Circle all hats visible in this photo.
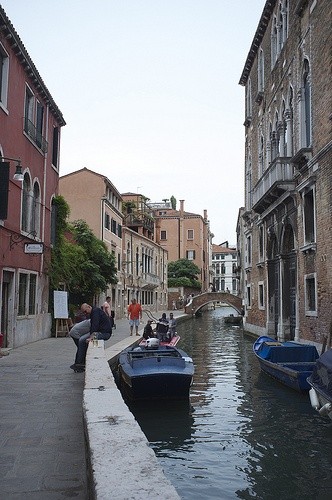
[130,298,136,301]
[148,320,153,322]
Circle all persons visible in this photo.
[70,319,90,372]
[126,299,143,336]
[143,312,176,350]
[70,303,112,372]
[170,285,233,310]
[103,296,114,337]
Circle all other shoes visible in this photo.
[70,364,86,373]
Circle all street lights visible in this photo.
[0,153,25,182]
[121,260,143,317]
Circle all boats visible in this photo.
[224,313,242,324]
[252,333,321,392]
[305,346,332,421]
[117,337,195,397]
[138,330,181,347]
